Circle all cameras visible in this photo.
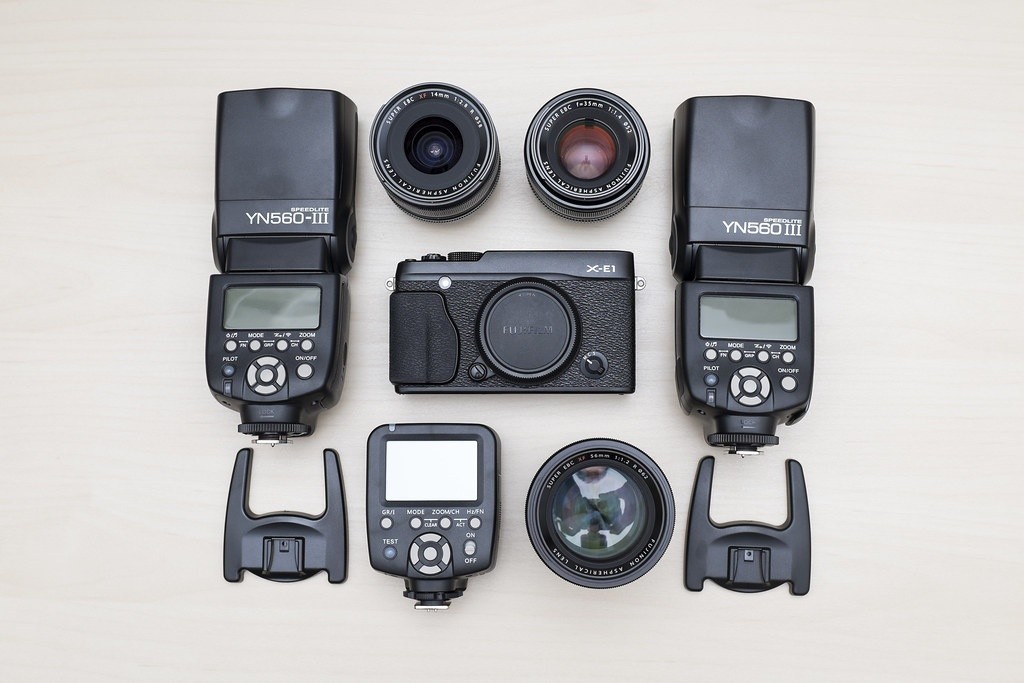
[387,251,639,396]
